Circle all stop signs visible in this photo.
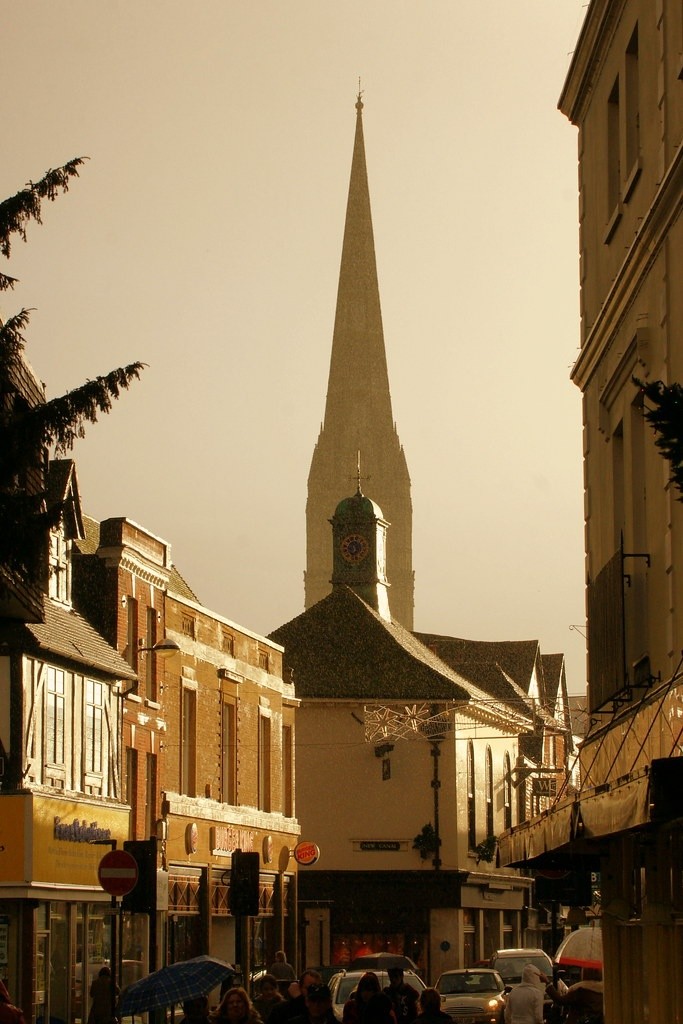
[97,850,138,896]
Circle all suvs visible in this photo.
[434,969,513,1024]
[328,967,448,1024]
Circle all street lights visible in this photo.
[90,839,118,1024]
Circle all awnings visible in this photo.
[495,755,683,869]
[553,926,603,971]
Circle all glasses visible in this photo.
[359,986,376,993]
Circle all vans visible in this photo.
[489,948,554,1018]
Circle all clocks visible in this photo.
[341,535,370,563]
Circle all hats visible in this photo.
[359,973,378,987]
[307,983,330,999]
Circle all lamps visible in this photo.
[139,638,180,657]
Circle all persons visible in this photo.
[503,963,604,1024]
[478,975,492,989]
[87,967,122,1024]
[454,978,468,989]
[179,949,342,1024]
[342,972,456,1024]
[0,977,27,1024]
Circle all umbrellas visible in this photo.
[116,955,235,1018]
[347,952,419,990]
